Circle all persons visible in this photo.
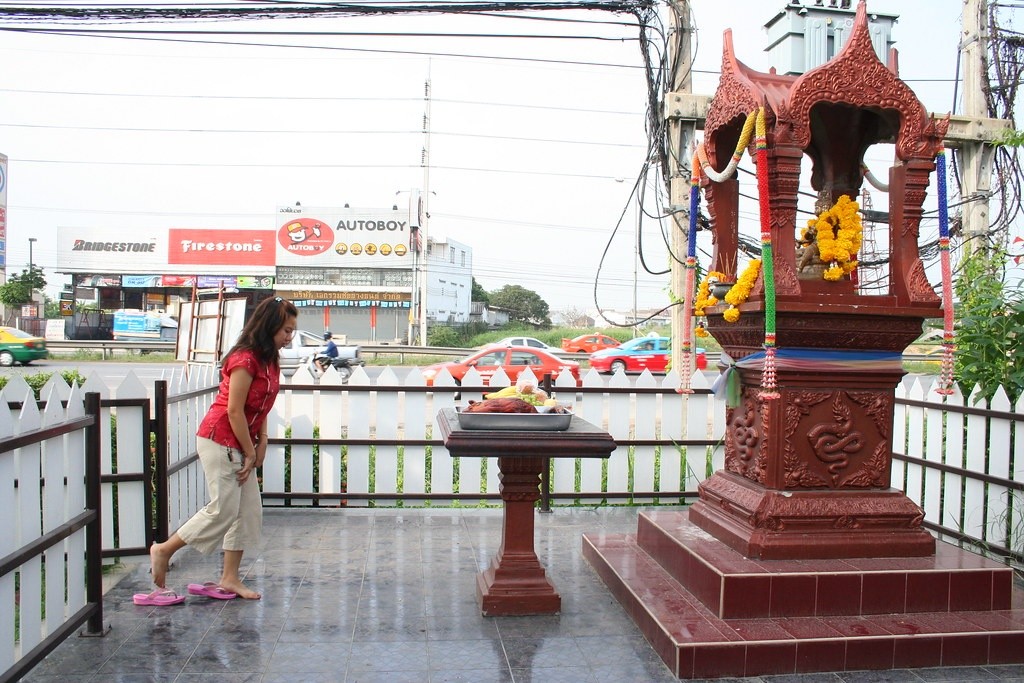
[494,352,505,365]
[637,343,652,351]
[150,297,298,599]
[313,331,338,376]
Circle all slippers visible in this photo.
[133,587,186,606]
[187,581,237,599]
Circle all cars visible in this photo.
[561,332,623,363]
[590,332,708,376]
[277,329,359,370]
[478,334,567,365]
[420,342,583,403]
[0,325,50,367]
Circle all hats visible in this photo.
[324,332,330,339]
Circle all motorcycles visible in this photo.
[295,347,357,384]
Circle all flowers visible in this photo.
[801,194,864,281]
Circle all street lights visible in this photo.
[29,238,38,300]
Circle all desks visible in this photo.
[436,408,617,616]
